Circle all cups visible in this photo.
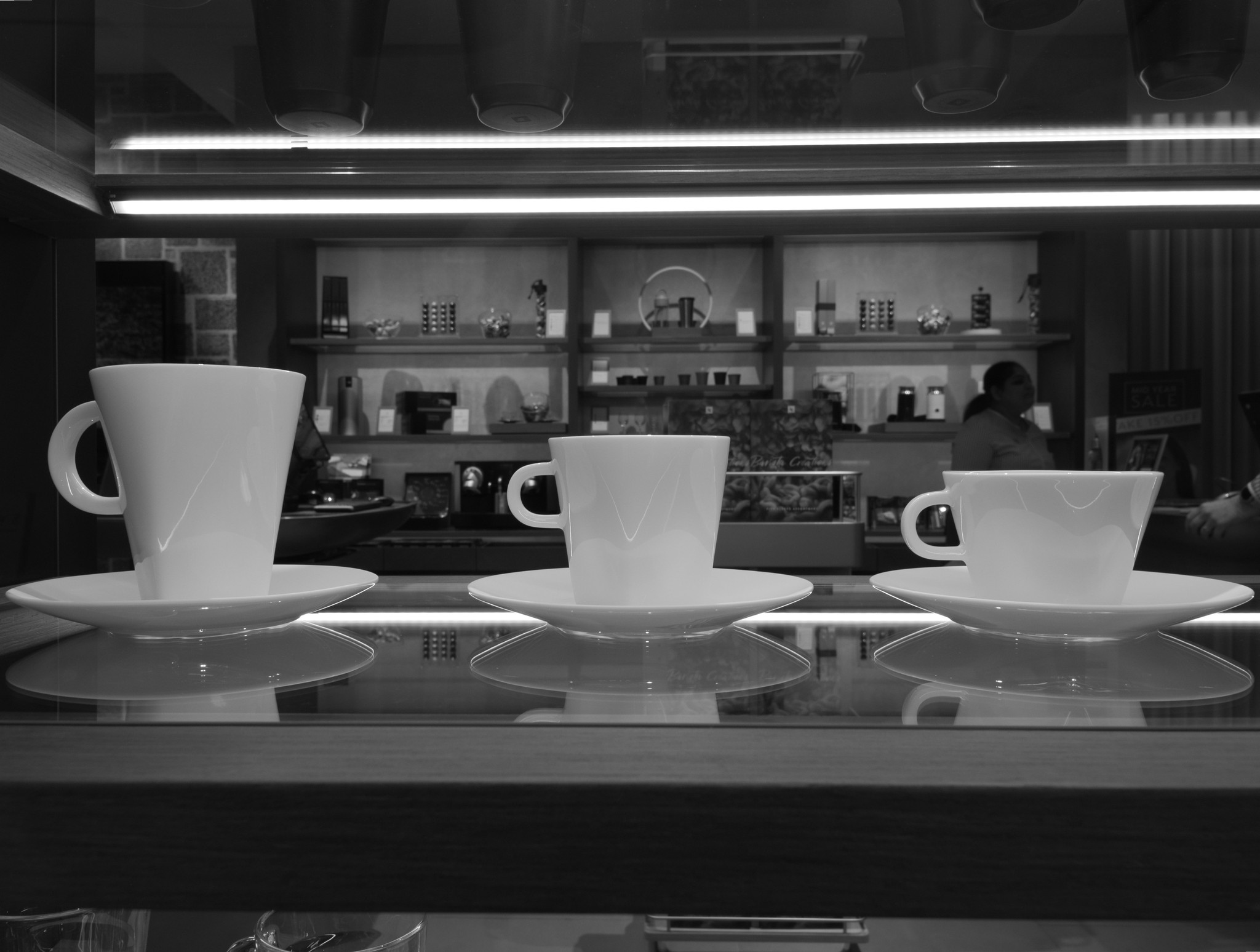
[46,364,306,603]
[93,688,283,724]
[616,372,740,386]
[900,469,1163,604]
[506,438,730,608]
[511,691,721,724]
[901,682,1147,727]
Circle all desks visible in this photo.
[0,582,1260,951]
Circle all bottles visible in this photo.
[927,386,944,420]
[897,385,914,420]
[337,376,360,436]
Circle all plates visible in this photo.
[872,624,1254,702]
[469,627,816,699]
[6,620,375,704]
[869,566,1255,636]
[5,563,380,639]
[466,569,814,639]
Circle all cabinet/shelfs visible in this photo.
[280,240,1077,528]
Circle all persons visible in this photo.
[951,361,1054,473]
[1185,474,1260,540]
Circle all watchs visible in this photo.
[1240,484,1257,505]
